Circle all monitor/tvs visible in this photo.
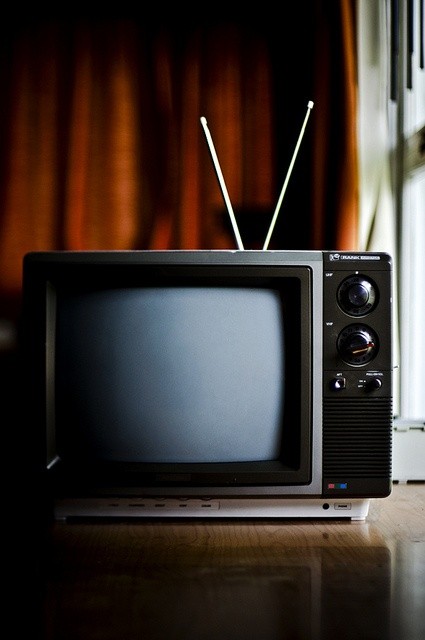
[23,101,395,523]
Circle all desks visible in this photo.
[0,518,392,639]
